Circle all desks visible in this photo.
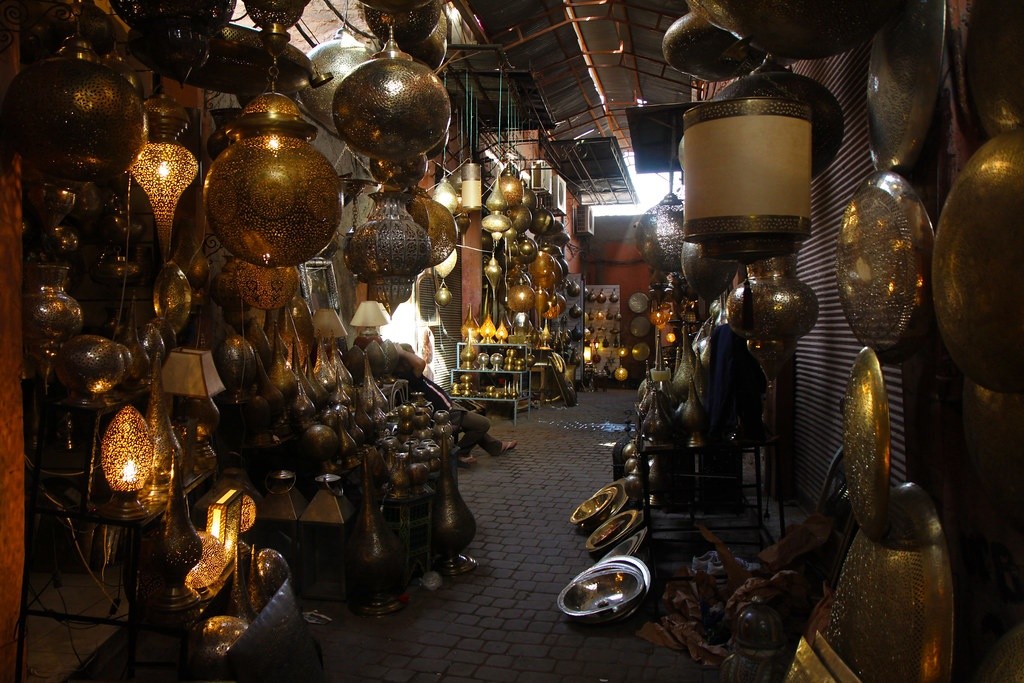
[643,431,781,564]
[16,462,214,682]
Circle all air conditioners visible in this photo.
[545,173,566,217]
[574,204,595,236]
[531,160,554,196]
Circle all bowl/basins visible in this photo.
[557,478,651,622]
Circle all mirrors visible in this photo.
[298,257,348,358]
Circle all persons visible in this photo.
[394,344,517,469]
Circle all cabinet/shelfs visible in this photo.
[451,342,534,427]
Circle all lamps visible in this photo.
[633,1,1024,683]
[484,73,631,382]
[0,0,481,682]
